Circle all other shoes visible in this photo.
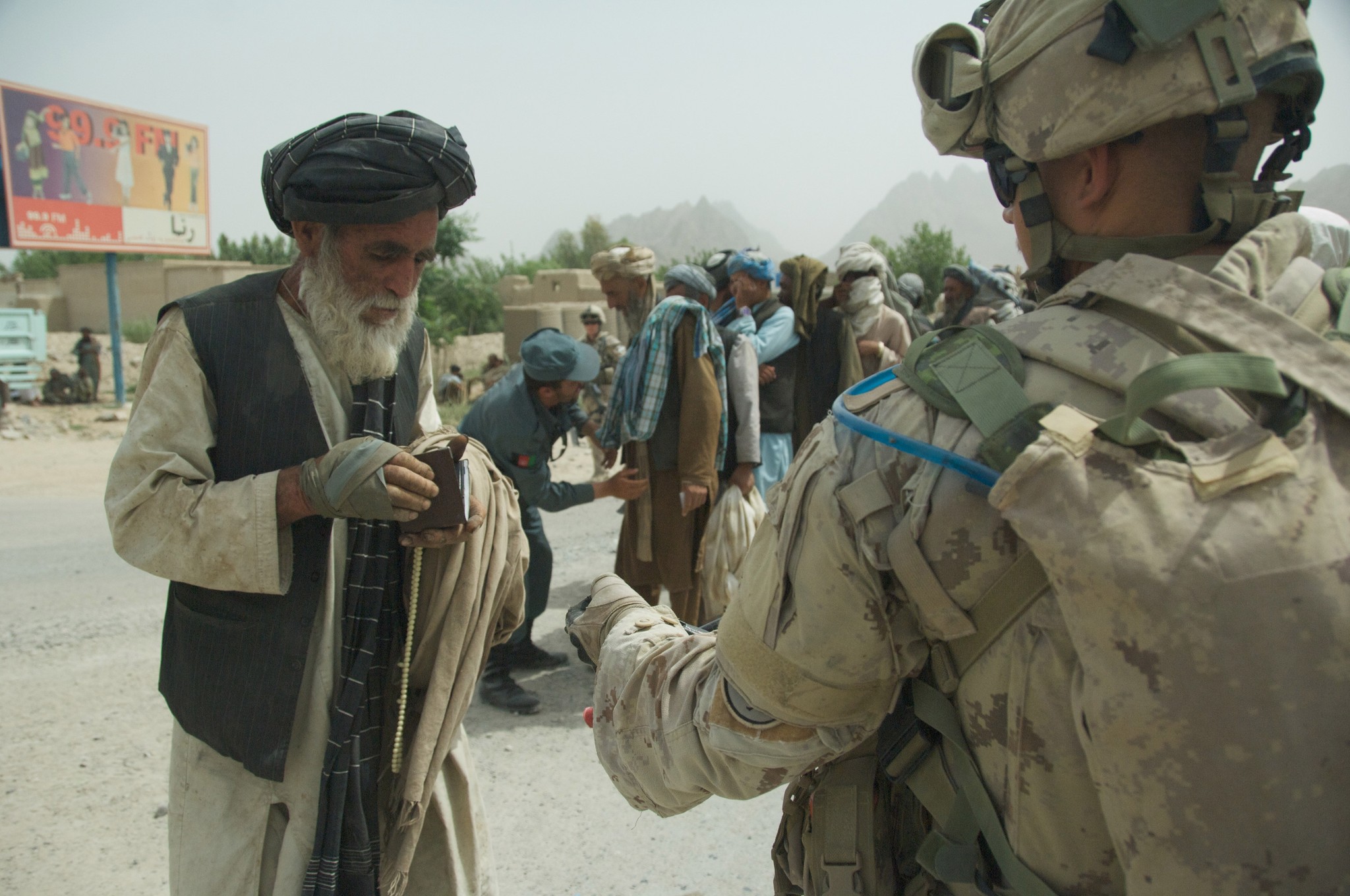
[507,640,570,672]
[478,678,543,716]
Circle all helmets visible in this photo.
[913,0,1325,164]
[579,305,606,326]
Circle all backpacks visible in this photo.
[894,312,1350,896]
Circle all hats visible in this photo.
[944,261,980,288]
[260,110,477,239]
[665,263,718,299]
[520,326,602,383]
[590,245,655,279]
[727,249,774,281]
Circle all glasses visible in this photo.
[582,313,598,319]
[982,137,1034,208]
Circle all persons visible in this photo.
[583,240,1044,626]
[70,327,102,403]
[562,0,1349,896]
[103,137,503,896]
[42,368,75,406]
[457,327,650,714]
[434,353,508,408]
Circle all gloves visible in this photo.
[563,572,650,673]
